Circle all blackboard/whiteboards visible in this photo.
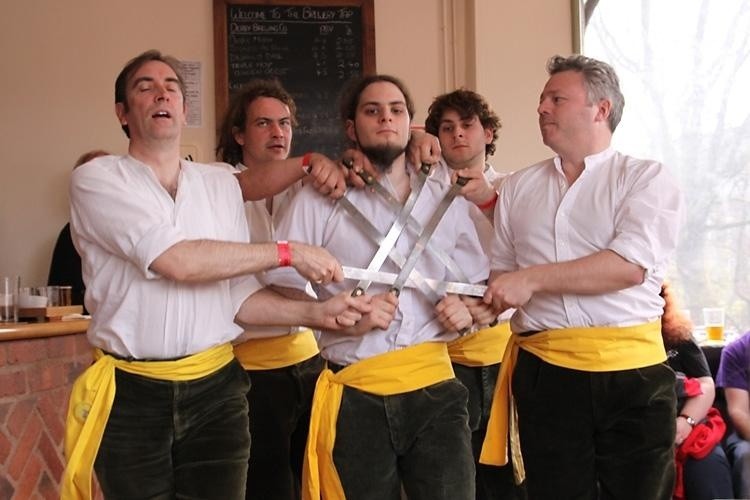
[214,1,377,166]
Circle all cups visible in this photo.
[47,286,59,306]
[1,275,20,323]
[60,286,71,305]
[705,308,725,340]
[31,288,39,296]
[24,287,31,296]
[39,287,47,296]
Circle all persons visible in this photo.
[68,50,372,500]
[47,149,112,316]
[263,76,491,500]
[215,81,379,500]
[715,329,750,499]
[462,54,683,500]
[410,89,514,494]
[660,284,732,499]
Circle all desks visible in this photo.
[0,316,105,500]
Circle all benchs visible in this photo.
[701,345,726,410]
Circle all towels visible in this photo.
[62,313,92,320]
